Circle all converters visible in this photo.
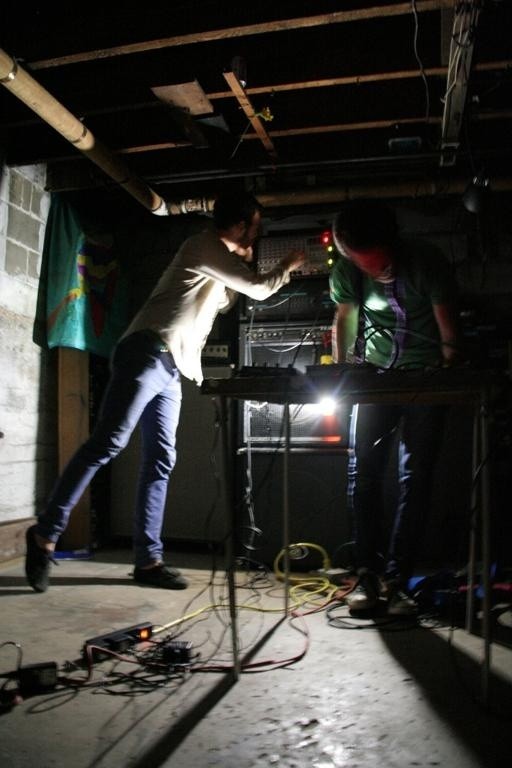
[16,660,57,694]
[162,640,194,663]
[315,567,351,586]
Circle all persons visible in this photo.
[319,195,464,616]
[20,187,308,598]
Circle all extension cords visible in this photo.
[83,621,153,659]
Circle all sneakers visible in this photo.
[26,524,58,592]
[348,585,418,614]
[134,563,186,590]
